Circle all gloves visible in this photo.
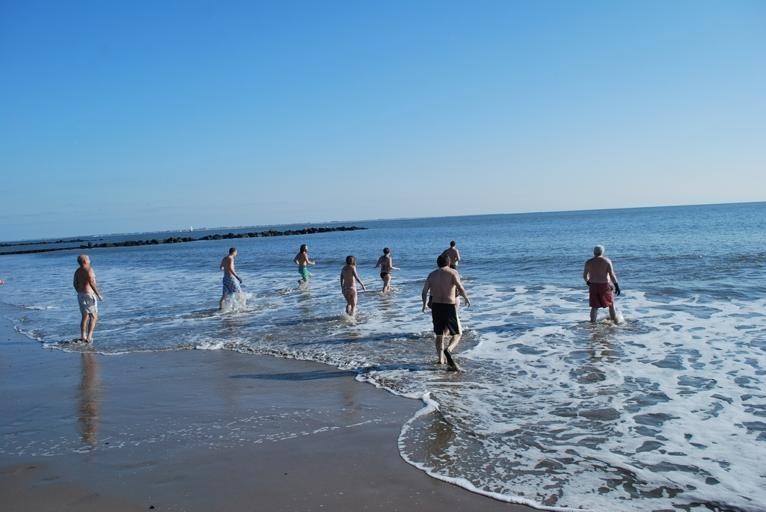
[614,283,620,296]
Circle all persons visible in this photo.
[76,346,106,448]
[583,244,621,323]
[440,241,460,270]
[449,263,464,311]
[73,254,104,344]
[293,243,316,286]
[374,247,401,293]
[220,247,242,310]
[421,253,470,372]
[339,255,366,319]
